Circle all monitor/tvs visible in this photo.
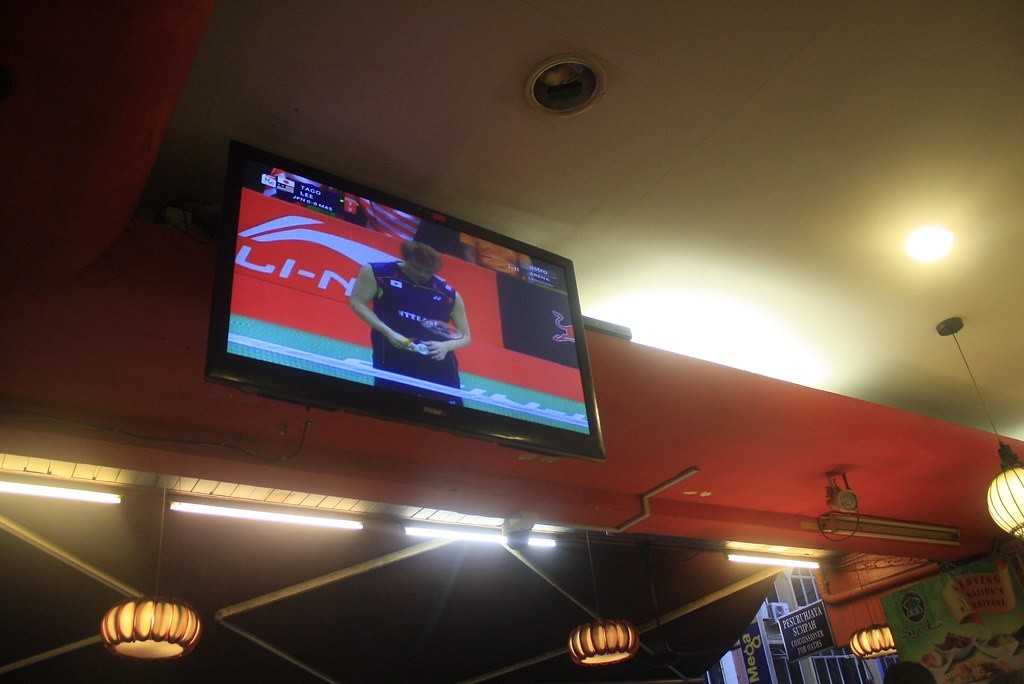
[203,140,607,463]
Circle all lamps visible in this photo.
[100,473,202,662]
[846,551,898,662]
[936,315,1024,540]
[567,528,638,670]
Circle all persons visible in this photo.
[348,238,470,409]
[457,230,536,290]
